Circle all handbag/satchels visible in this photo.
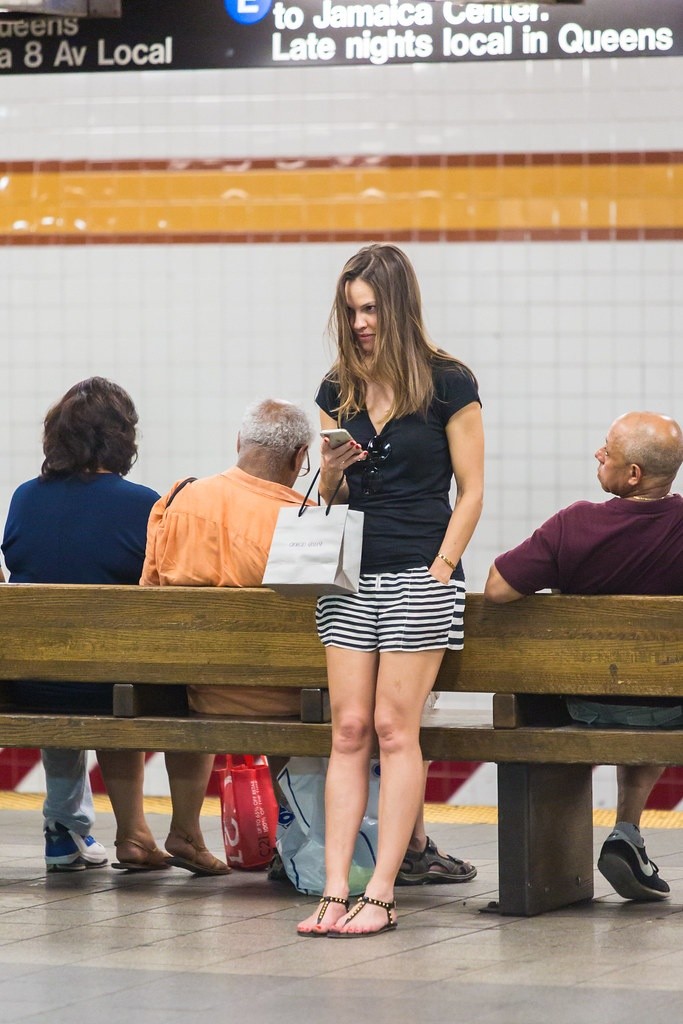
[215,752,279,872]
[260,466,365,595]
[275,756,380,898]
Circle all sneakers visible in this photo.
[598,830,670,900]
[44,822,108,870]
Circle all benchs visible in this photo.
[0,582,683,922]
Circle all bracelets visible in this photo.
[436,553,457,571]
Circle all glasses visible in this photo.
[361,435,392,494]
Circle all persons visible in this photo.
[484,411,682,900]
[138,398,477,885]
[0,376,232,876]
[296,244,484,939]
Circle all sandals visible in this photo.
[396,833,477,883]
[113,836,176,870]
[164,825,233,875]
[297,898,398,939]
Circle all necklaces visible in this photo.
[634,492,671,501]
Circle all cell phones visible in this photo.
[320,429,363,456]
